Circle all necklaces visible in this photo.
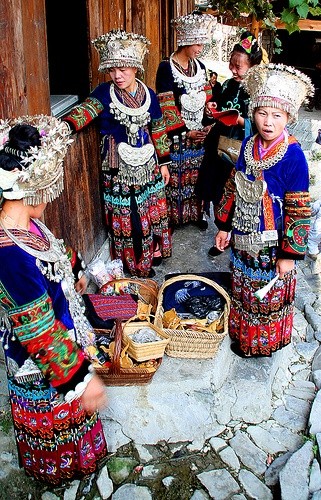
[232,130,288,234]
[170,51,205,131]
[0,207,89,344]
[109,78,155,185]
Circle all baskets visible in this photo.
[121,314,170,362]
[154,275,231,359]
[98,278,159,325]
[92,317,163,387]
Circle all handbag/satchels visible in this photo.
[217,117,254,165]
[81,292,141,329]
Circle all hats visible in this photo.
[239,63,315,127]
[0,113,75,206]
[170,13,217,46]
[91,30,151,74]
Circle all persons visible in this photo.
[193,31,263,258]
[154,11,218,236]
[209,72,221,99]
[60,29,173,278]
[0,114,107,486]
[214,63,312,358]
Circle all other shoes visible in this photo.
[146,268,156,278]
[208,244,230,258]
[198,219,208,232]
